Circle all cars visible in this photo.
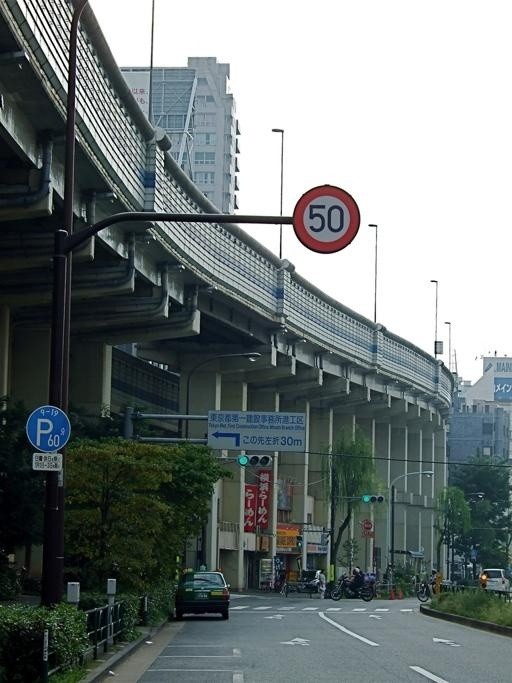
[174,570,231,618]
[297,570,326,593]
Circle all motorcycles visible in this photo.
[330,573,375,601]
[415,581,434,602]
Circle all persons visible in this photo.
[430,569,438,594]
[347,566,363,597]
[481,570,489,593]
[318,569,326,600]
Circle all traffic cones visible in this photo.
[383,590,403,600]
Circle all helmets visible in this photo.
[353,567,360,575]
[431,568,437,574]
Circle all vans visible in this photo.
[479,568,510,593]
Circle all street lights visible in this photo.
[430,280,450,370]
[272,129,284,259]
[387,470,434,568]
[368,224,377,322]
[452,492,485,582]
[185,352,263,438]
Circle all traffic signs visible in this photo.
[292,185,361,254]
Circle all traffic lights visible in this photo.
[236,455,273,468]
[361,494,385,504]
